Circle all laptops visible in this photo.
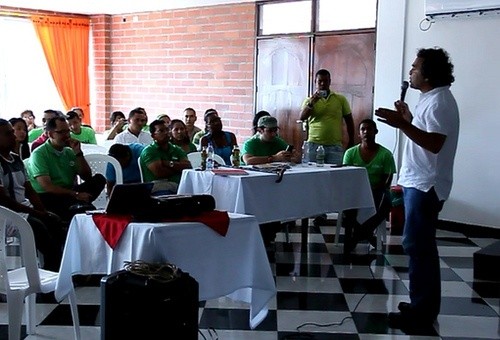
[86,182,154,215]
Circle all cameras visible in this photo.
[317,90,327,97]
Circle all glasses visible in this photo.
[54,129,70,135]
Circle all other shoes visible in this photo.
[369,235,384,249]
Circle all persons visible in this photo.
[0,106,301,274]
[375,46,460,331]
[300,69,354,165]
[342,119,396,259]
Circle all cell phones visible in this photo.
[286,144,294,152]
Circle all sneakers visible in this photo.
[398,302,438,320]
[389,312,439,336]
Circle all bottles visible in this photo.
[232,145,240,168]
[201,146,206,171]
[302,141,310,167]
[315,146,325,168]
[206,142,214,170]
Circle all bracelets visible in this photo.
[75,192,79,199]
[307,105,312,110]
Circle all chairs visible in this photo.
[0,204,83,340]
[137,156,167,200]
[334,207,387,251]
[186,152,226,169]
[76,153,125,210]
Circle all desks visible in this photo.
[52,208,277,340]
[174,160,379,270]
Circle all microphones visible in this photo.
[401,80,408,101]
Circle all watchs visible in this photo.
[169,161,174,168]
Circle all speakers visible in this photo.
[99,270,199,340]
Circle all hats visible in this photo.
[257,116,280,130]
